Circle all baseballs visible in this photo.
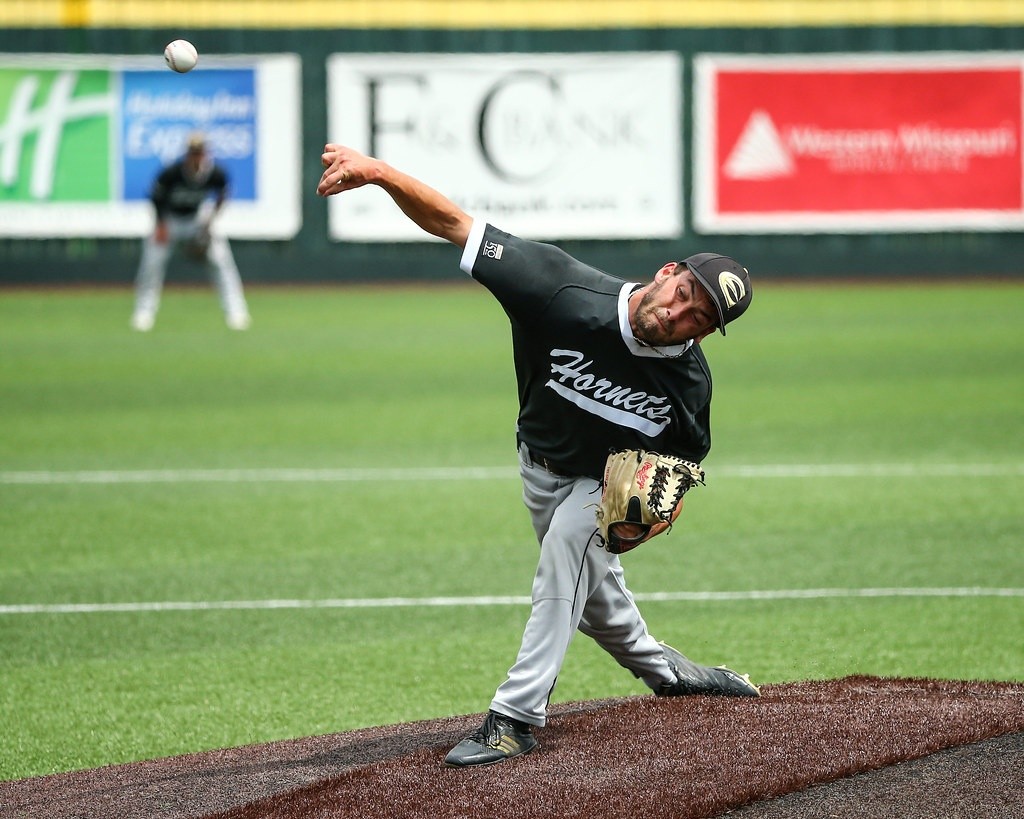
[164,39,199,74]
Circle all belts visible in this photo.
[517,432,604,476]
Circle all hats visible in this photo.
[680,253,752,336]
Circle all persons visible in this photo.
[134,138,249,329]
[317,144,763,766]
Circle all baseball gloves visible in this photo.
[582,447,708,555]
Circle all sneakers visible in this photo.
[654,640,761,697]
[444,710,538,767]
